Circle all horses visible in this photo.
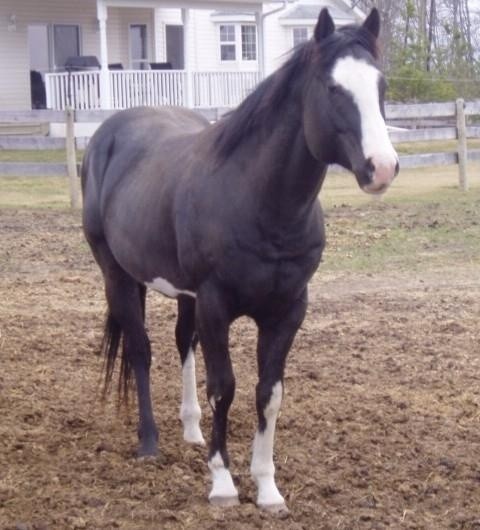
[81,6,400,520]
